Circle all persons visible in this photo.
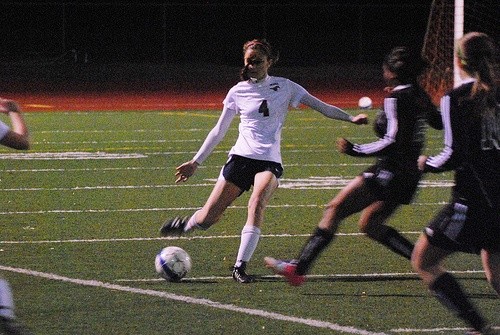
[263,49,444,286]
[0,100,29,335]
[160,39,368,283]
[411,32,500,335]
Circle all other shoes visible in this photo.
[0,316,29,335]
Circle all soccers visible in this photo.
[358,96,373,109]
[154,246,192,282]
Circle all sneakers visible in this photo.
[232,260,254,283]
[160,216,186,233]
[264,255,304,286]
